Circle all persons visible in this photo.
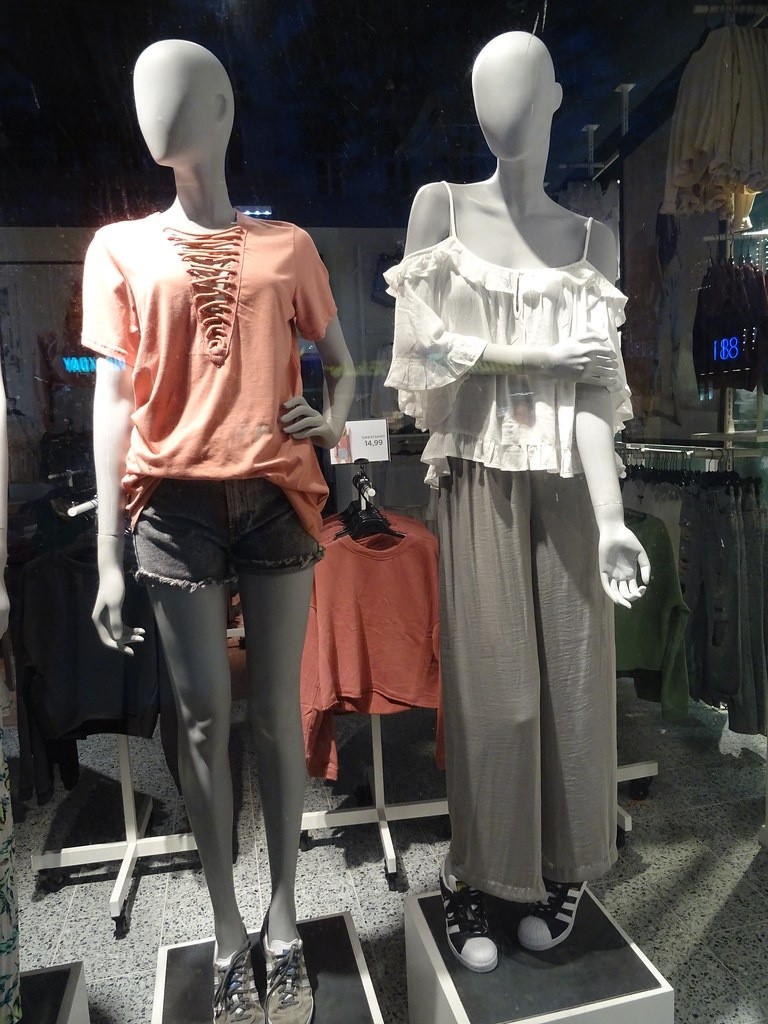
[383,30,652,974]
[79,39,357,1024]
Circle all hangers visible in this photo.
[333,476,405,542]
[703,232,764,279]
[692,0,768,40]
[618,448,762,491]
[41,470,137,560]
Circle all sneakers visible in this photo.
[518,871,588,951]
[259,905,313,1024]
[210,920,265,1024]
[439,857,497,973]
